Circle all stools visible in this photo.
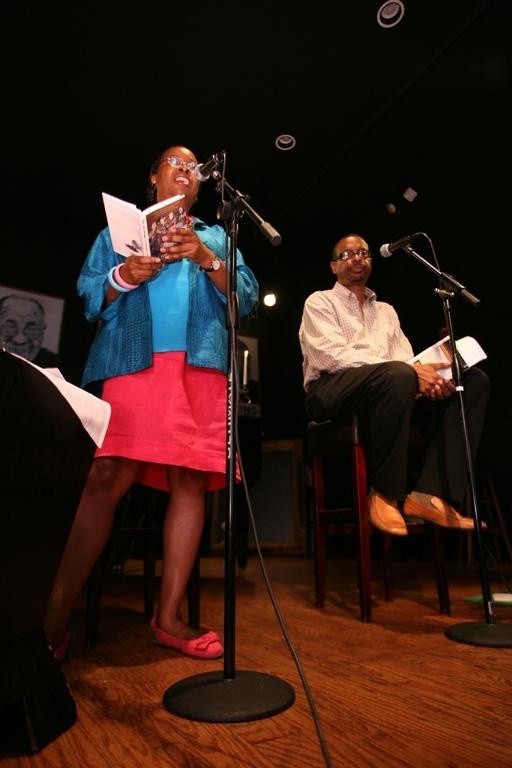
[303,410,454,624]
[79,509,204,644]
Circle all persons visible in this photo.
[44,145,259,659]
[0,294,47,361]
[298,232,489,538]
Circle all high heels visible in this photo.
[49,606,85,661]
[150,616,224,659]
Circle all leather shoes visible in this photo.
[368,491,408,537]
[404,493,486,531]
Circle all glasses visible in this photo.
[158,157,200,172]
[334,249,372,261]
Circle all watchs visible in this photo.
[199,254,221,273]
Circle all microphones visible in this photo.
[194,153,221,183]
[379,236,412,258]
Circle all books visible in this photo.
[414,336,465,398]
[98,190,188,266]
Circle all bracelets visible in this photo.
[109,266,131,293]
[114,263,139,290]
